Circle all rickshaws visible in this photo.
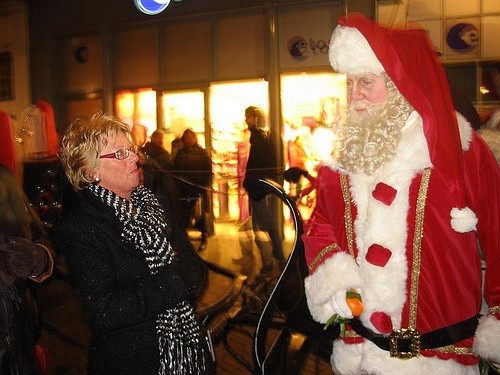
[0,100,317,374]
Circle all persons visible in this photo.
[288,133,312,220]
[0,163,56,375]
[301,12,500,375]
[141,128,212,249]
[232,107,286,271]
[57,117,219,375]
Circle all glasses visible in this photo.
[99,144,138,160]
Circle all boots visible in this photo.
[198,213,209,251]
